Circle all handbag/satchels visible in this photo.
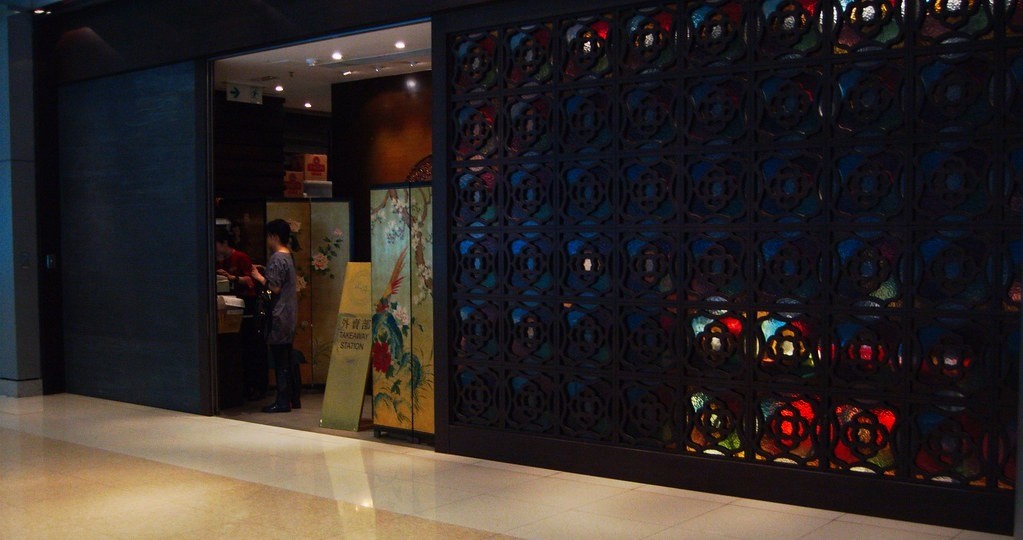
[238,281,281,336]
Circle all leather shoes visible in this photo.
[290,399,301,408]
[262,403,291,412]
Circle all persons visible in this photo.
[251,219,302,413]
[214,226,256,289]
